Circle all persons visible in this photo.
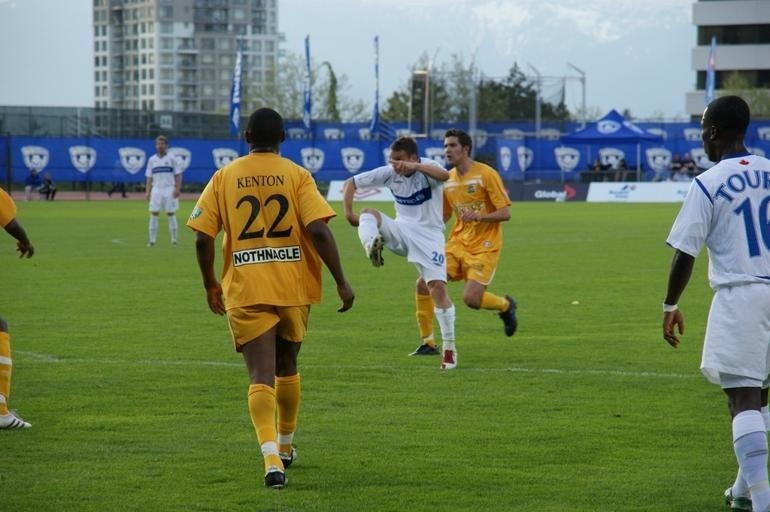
[409,126,519,359]
[0,183,37,430]
[341,135,461,373]
[190,104,355,493]
[38,172,58,201]
[142,133,184,248]
[107,181,132,199]
[589,152,700,183]
[24,167,41,201]
[658,91,770,512]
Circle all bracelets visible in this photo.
[662,301,679,314]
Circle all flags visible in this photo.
[225,34,244,137]
[703,36,718,104]
[301,33,314,130]
[368,36,381,137]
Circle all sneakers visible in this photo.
[724,487,753,511]
[368,235,385,266]
[0,412,31,429]
[409,344,439,356]
[441,350,457,369]
[499,295,517,336]
[264,447,297,489]
